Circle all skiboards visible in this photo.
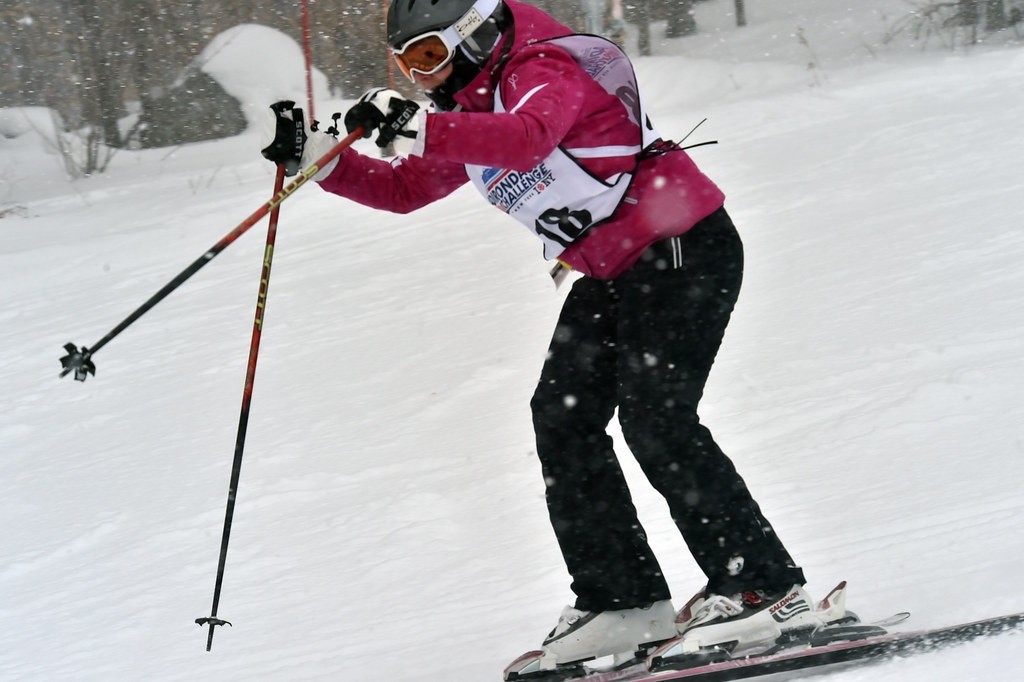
[501,577,1024,682]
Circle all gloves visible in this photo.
[344,86,420,160]
[260,98,340,181]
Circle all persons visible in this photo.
[259,1,815,666]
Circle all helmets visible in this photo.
[387,1,508,65]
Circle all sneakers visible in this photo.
[680,582,816,645]
[541,598,677,663]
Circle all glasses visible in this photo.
[392,32,453,83]
